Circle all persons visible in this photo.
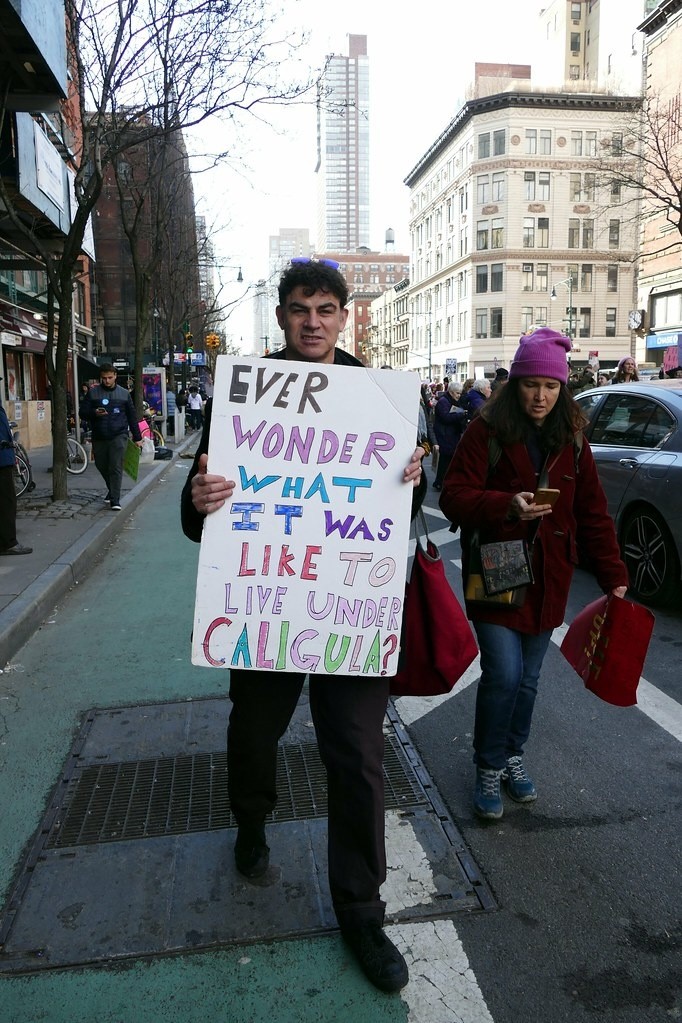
[437,329,629,819]
[166,384,209,437]
[416,368,511,492]
[80,382,92,431]
[78,362,143,511]
[0,404,34,556]
[180,262,428,991]
[565,358,610,410]
[608,357,641,386]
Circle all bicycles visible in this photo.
[140,412,165,454]
[65,435,89,475]
[8,421,37,498]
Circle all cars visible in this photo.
[564,379,682,607]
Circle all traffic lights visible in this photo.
[206,335,211,347]
[210,334,216,350]
[215,336,220,346]
[361,344,367,353]
[185,334,194,354]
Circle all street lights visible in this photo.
[549,268,582,371]
[151,288,162,367]
[179,263,245,391]
[396,307,433,383]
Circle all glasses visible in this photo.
[290,256,340,271]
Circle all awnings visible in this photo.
[0,306,81,359]
[77,353,101,379]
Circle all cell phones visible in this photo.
[532,489,560,507]
[99,408,106,413]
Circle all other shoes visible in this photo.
[432,482,442,490]
[105,491,121,510]
[1,542,33,555]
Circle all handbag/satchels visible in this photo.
[463,539,533,606]
[396,538,480,697]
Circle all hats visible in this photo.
[618,356,637,370]
[508,328,572,383]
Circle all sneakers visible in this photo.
[474,760,503,820]
[234,817,269,878]
[504,755,538,802]
[347,929,409,991]
